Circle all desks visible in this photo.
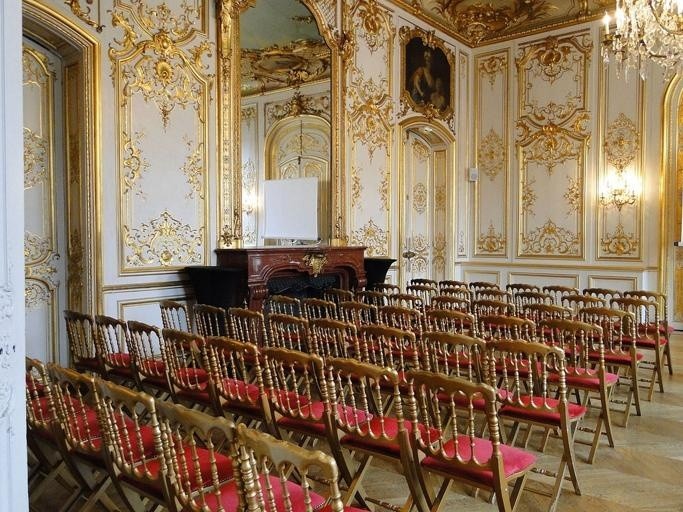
[178,246,398,347]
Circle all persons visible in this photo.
[408,50,435,105]
[428,78,446,112]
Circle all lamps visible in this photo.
[600,0,683,81]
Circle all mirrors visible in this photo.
[216,0,349,250]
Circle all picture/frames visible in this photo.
[398,24,456,121]
